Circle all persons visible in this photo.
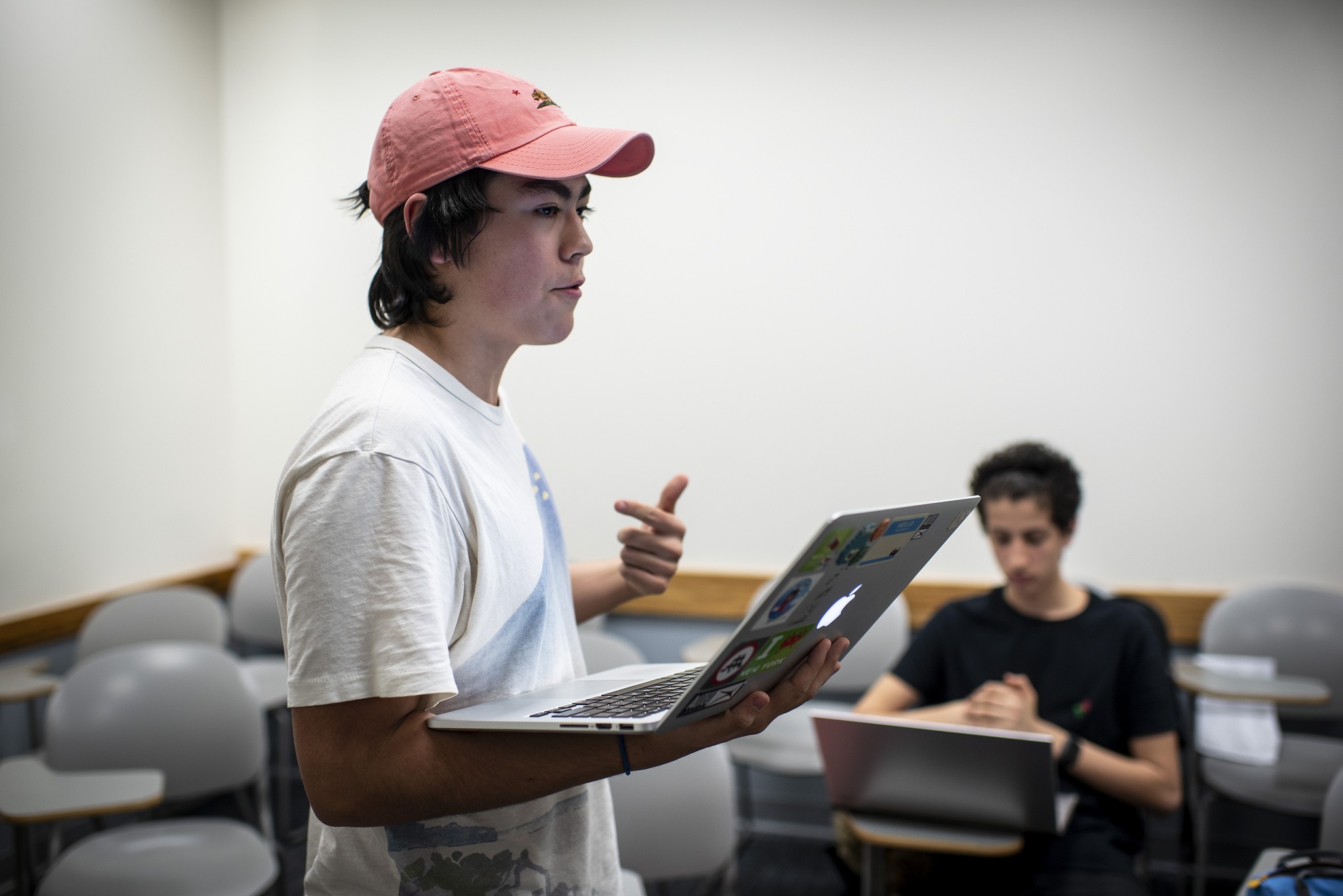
[271,65,851,895]
[853,443,1184,896]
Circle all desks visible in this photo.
[0,653,58,750]
[830,802,1024,896]
[0,754,168,896]
[1173,658,1333,706]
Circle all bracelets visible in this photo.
[1054,732,1080,772]
[617,734,631,775]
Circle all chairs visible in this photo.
[735,578,912,847]
[226,554,294,710]
[1182,583,1343,896]
[75,584,231,668]
[578,608,742,896]
[31,643,283,896]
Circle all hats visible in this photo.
[367,67,655,228]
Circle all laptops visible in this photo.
[811,710,1079,834]
[428,494,980,731]
